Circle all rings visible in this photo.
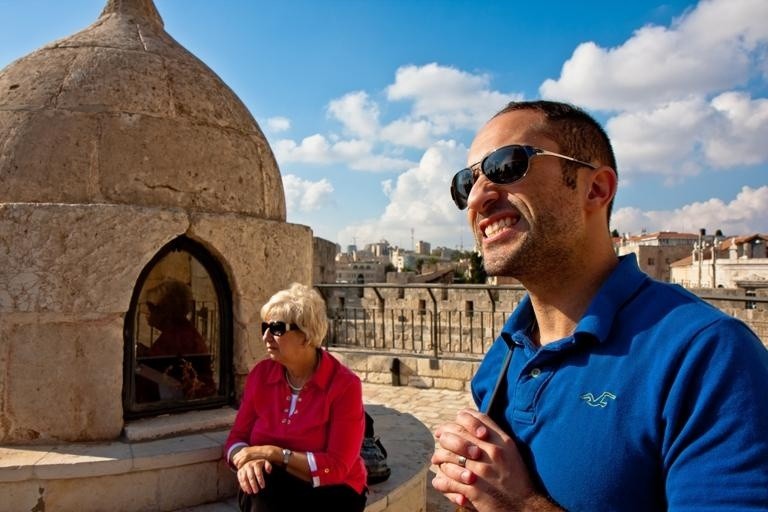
[457,455,468,470]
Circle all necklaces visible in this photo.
[285,352,321,391]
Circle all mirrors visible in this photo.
[122,234,234,419]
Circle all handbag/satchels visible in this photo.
[360,435,391,486]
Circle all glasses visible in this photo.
[261,319,299,337]
[145,300,166,313]
[450,142,599,214]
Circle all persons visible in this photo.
[430,100,768,512]
[136,278,218,406]
[222,280,371,512]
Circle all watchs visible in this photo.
[279,448,291,468]
